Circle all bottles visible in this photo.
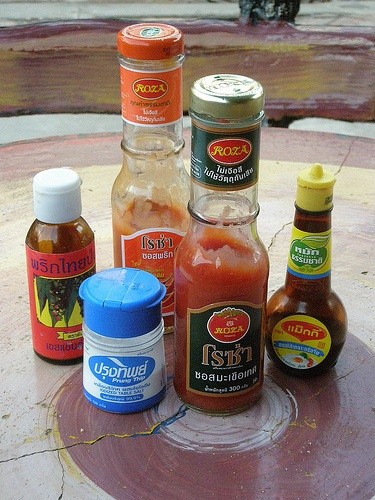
[265,162,348,378]
[110,23,191,336]
[24,168,96,364]
[173,72,270,415]
